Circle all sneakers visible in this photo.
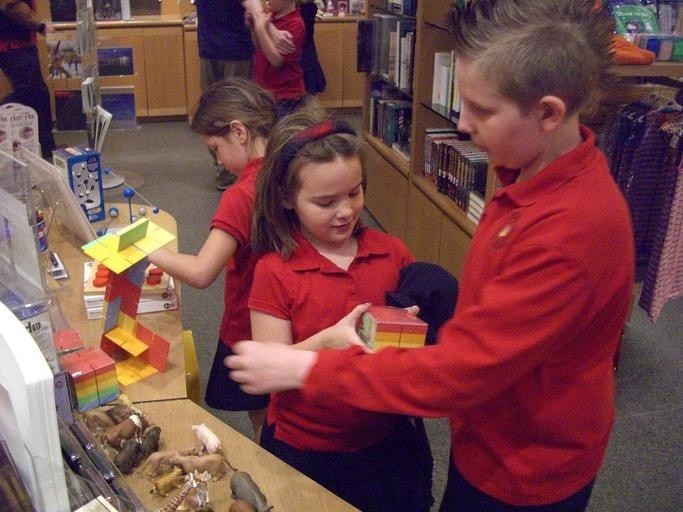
[216,168,237,190]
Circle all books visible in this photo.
[366,0,494,230]
[0,301,73,512]
[74,0,113,159]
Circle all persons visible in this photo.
[192,0,328,193]
[221,1,637,511]
[0,1,58,165]
[146,80,283,441]
[247,103,434,511]
